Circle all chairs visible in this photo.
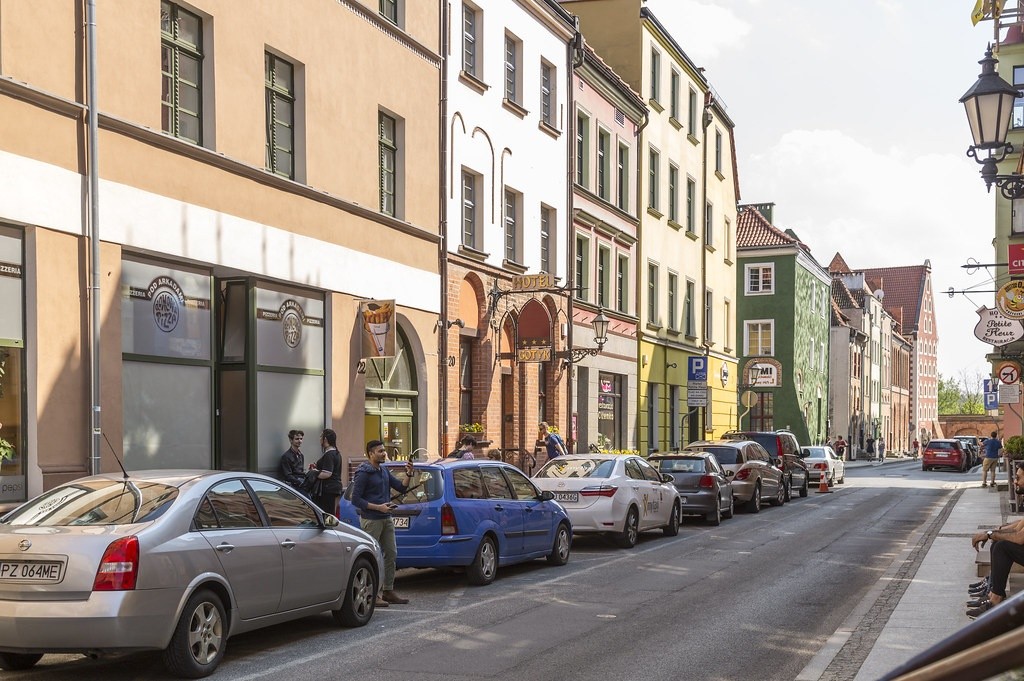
[424,477,435,497]
[661,459,673,470]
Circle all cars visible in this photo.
[800,445,845,487]
[0,469,383,680]
[646,452,734,527]
[335,457,574,585]
[529,453,683,549]
[922,438,969,472]
[680,439,786,514]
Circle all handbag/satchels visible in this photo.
[555,435,568,455]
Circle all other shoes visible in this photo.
[991,483,996,487]
[967,596,989,609]
[970,589,990,598]
[382,592,409,604]
[969,576,990,589]
[966,600,994,620]
[982,483,987,487]
[375,595,390,607]
[968,582,988,593]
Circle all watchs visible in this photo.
[986,531,992,539]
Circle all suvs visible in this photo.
[721,429,810,502]
[952,436,989,471]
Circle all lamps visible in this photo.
[737,358,762,391]
[561,301,611,369]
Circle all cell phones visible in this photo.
[407,454,414,468]
[389,505,398,509]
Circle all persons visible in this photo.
[966,462,1024,617]
[922,439,928,451]
[539,422,570,457]
[878,438,885,461]
[826,437,832,446]
[969,517,1024,594]
[977,432,1002,486]
[312,429,344,513]
[353,440,414,608]
[864,435,875,461]
[280,430,309,499]
[912,438,919,460]
[448,435,478,459]
[833,435,848,461]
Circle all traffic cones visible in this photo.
[815,464,834,493]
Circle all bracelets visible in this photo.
[405,471,414,477]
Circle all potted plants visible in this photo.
[538,424,559,441]
[458,422,484,441]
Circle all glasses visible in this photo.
[540,427,545,431]
